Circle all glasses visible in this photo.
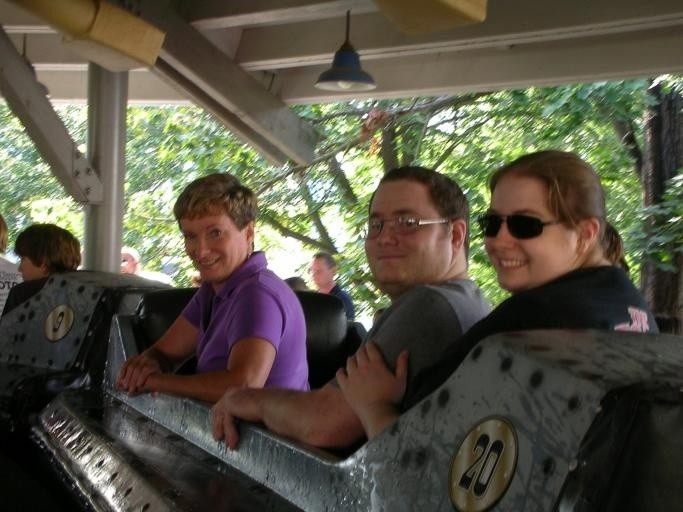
[360,217,448,238]
[479,214,564,240]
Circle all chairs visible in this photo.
[134,284,366,407]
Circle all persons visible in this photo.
[0,223,80,319]
[118,246,175,285]
[310,252,354,321]
[115,172,312,403]
[209,166,493,450]
[334,150,661,440]
[0,214,26,316]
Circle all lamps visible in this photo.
[314,9,378,92]
